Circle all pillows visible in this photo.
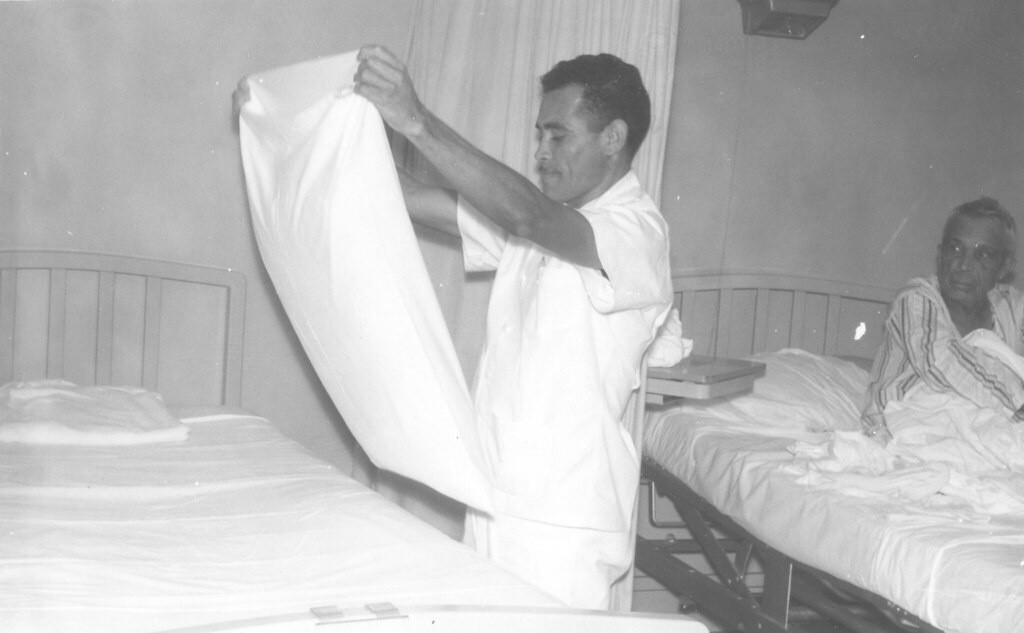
[235,48,507,520]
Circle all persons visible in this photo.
[862,197,1024,440]
[231,45,674,612]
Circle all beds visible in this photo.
[0,248,711,633]
[621,270,1023,633]
